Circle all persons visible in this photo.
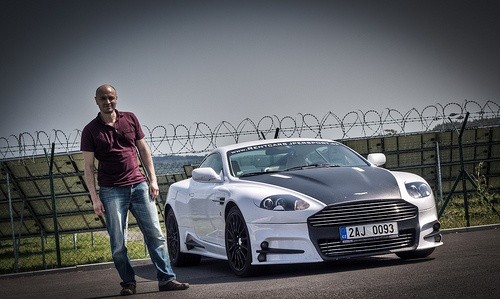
[80,83,190,296]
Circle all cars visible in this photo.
[164,138,443,277]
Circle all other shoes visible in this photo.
[119,281,137,296]
[158,279,191,292]
